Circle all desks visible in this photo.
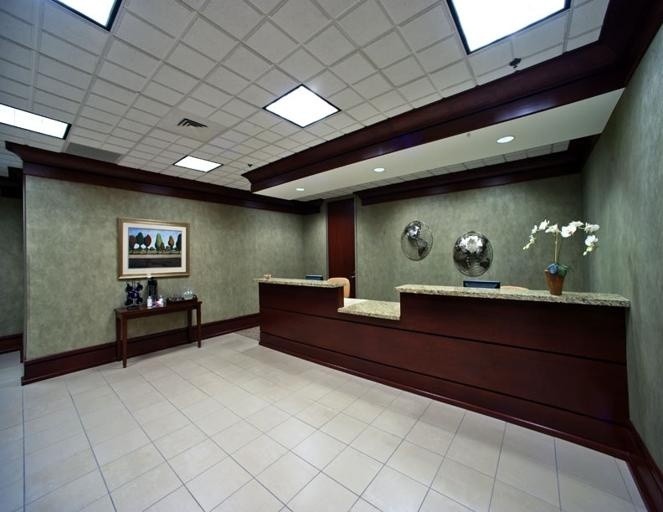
[114,296,202,368]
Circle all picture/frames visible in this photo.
[117,217,190,281]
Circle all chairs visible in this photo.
[501,285,530,291]
[327,277,350,298]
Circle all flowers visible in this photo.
[524,217,599,277]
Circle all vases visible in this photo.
[545,271,565,295]
